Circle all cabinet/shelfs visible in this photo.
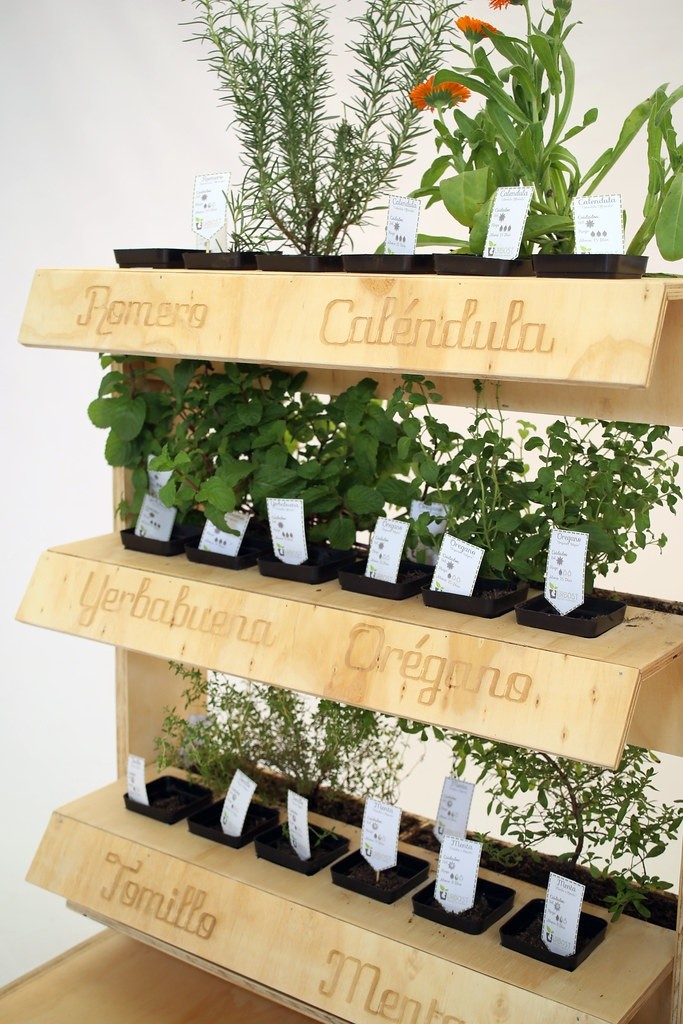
[18,267,683,1024]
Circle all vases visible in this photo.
[187,796,280,850]
[499,899,609,971]
[330,849,430,904]
[531,254,648,280]
[114,248,206,269]
[342,255,433,274]
[412,876,516,935]
[255,820,352,876]
[124,775,213,825]
[432,252,536,277]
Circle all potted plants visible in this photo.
[183,166,291,271]
[176,0,467,272]
[150,659,419,846]
[87,351,683,639]
[405,719,683,932]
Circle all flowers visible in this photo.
[373,0,683,261]
